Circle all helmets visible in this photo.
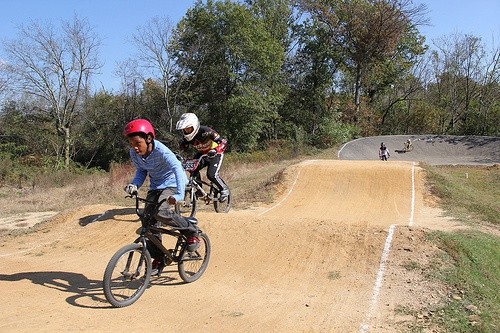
[381,142,385,147]
[176,113,200,141]
[123,119,155,138]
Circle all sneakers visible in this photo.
[190,191,203,198]
[220,186,229,202]
[187,224,200,252]
[152,259,161,271]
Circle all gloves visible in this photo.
[126,184,137,194]
[208,150,217,158]
[173,151,178,155]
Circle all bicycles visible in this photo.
[402,143,414,152]
[102,190,211,309]
[174,154,231,218]
[379,150,389,161]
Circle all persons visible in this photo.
[175,113,229,202]
[379,143,390,161]
[124,119,200,272]
[404,139,412,149]
